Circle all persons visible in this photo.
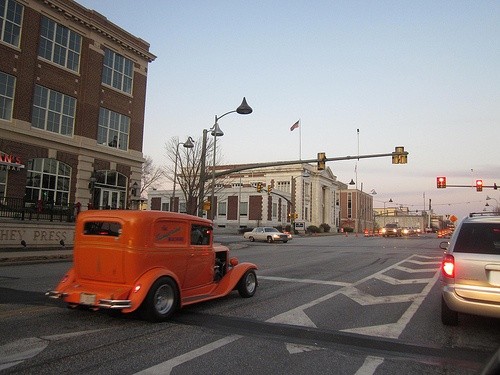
[109,136,121,148]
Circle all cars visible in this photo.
[243,227,292,243]
[46,209,259,324]
[401,227,422,236]
[382,224,402,238]
[439,213,500,327]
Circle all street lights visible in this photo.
[384,198,393,225]
[339,179,355,232]
[210,97,252,221]
[486,195,498,214]
[358,190,377,232]
[486,203,494,213]
[291,173,310,234]
[199,123,223,218]
[171,137,195,213]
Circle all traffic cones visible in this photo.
[346,232,348,237]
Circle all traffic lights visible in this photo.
[437,177,446,188]
[476,180,482,191]
[294,214,298,219]
[268,185,272,194]
[257,182,263,193]
